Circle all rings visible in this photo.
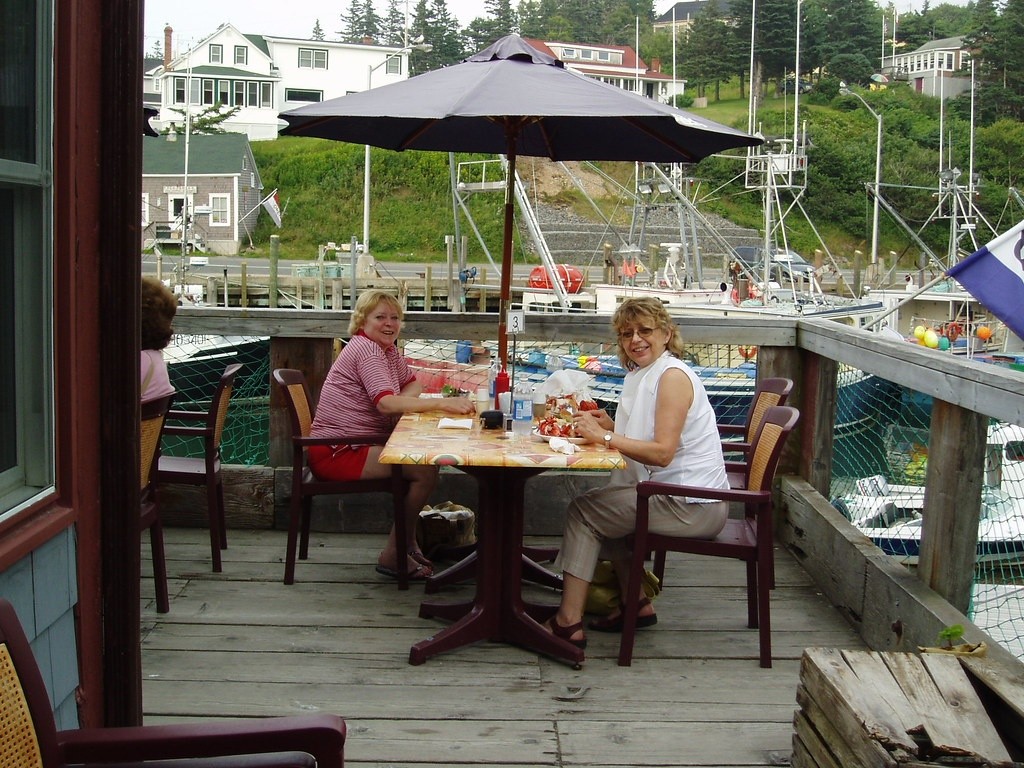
[574,421,578,428]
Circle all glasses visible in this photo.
[618,326,658,338]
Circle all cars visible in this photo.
[728,246,816,283]
[780,78,815,96]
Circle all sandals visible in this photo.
[588,598,657,630]
[550,615,587,648]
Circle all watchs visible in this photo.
[604,430,612,448]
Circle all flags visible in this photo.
[945,219,1024,340]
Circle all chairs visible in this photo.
[856,474,889,498]
[138,390,179,614]
[617,406,800,669]
[273,369,411,590]
[870,84,886,91]
[0,597,347,768]
[651,377,794,591]
[152,362,244,573]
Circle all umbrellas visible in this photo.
[871,74,888,82]
[278,35,766,361]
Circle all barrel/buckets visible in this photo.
[456,341,471,364]
[528,353,547,364]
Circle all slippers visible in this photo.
[407,552,433,567]
[375,564,431,579]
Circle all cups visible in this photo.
[476,389,491,416]
[533,393,546,419]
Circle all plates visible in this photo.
[533,428,591,444]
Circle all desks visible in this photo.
[378,392,627,670]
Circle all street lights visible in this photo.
[357,43,434,278]
[838,87,882,282]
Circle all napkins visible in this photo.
[437,418,474,429]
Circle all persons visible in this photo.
[307,289,475,580]
[538,298,729,650]
[140,277,177,401]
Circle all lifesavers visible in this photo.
[738,345,757,358]
[730,290,741,306]
[946,322,959,342]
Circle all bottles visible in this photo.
[505,414,513,431]
[572,343,580,355]
[512,374,532,435]
[488,358,502,411]
[495,369,510,411]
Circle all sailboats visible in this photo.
[523,0,1024,665]
